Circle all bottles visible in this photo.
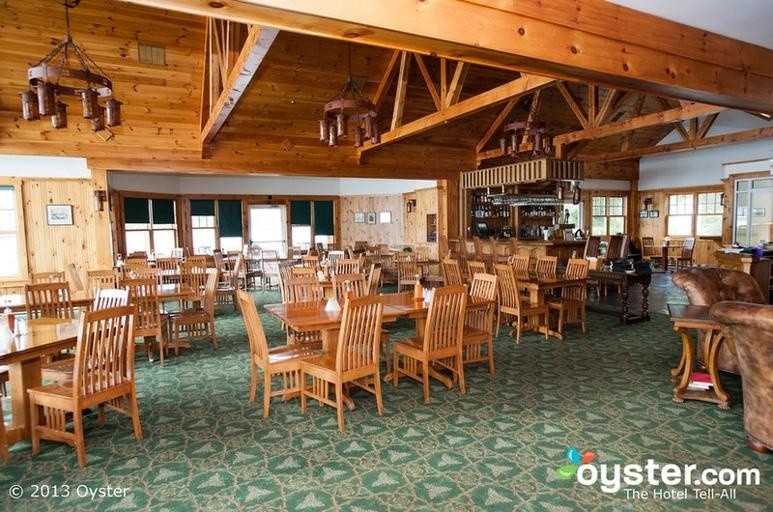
[3,308,14,335]
[755,244,763,257]
[470,190,557,218]
[345,279,352,300]
[499,224,539,238]
[544,226,549,241]
[325,298,341,312]
[413,274,423,302]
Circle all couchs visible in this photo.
[709,300,773,453]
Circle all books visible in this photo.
[687,372,713,392]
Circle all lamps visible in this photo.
[499,94,556,158]
[406,199,416,213]
[18,1,125,129]
[319,42,382,147]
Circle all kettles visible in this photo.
[574,229,582,240]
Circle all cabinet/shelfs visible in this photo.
[517,186,560,240]
[714,249,773,300]
[469,187,514,240]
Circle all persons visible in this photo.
[115,252,127,267]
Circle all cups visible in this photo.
[14,317,29,335]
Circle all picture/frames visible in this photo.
[355,213,366,222]
[368,212,376,224]
[46,204,74,226]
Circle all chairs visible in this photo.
[672,265,773,374]
